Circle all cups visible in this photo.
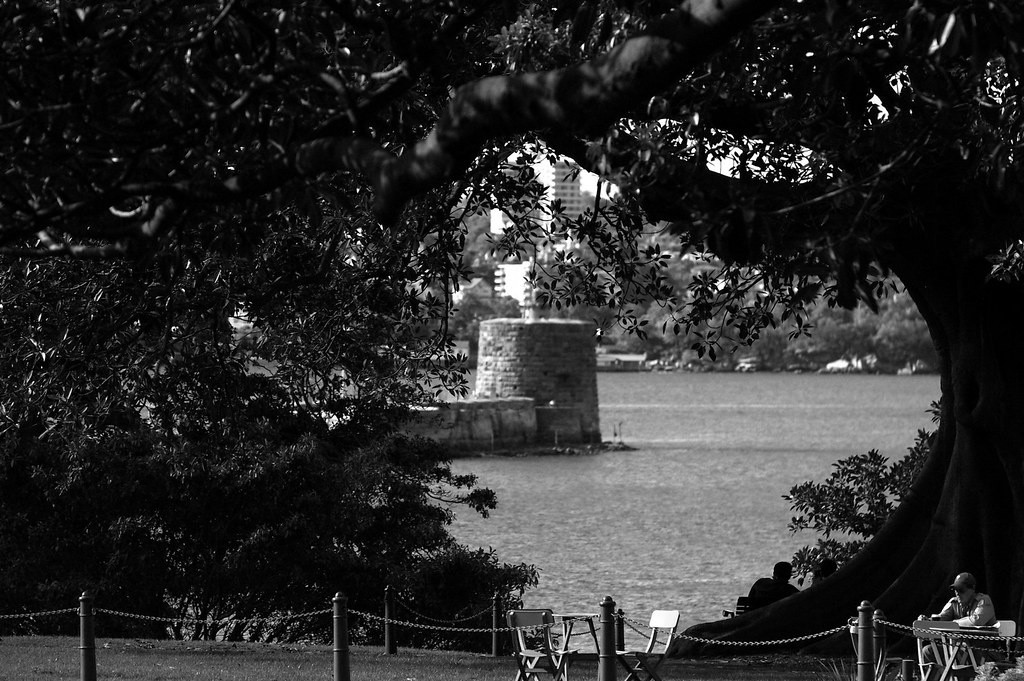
[931,614,941,621]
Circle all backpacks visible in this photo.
[747,577,783,611]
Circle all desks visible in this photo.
[551,612,601,681]
[930,628,996,681]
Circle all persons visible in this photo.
[813,559,837,584]
[747,562,799,611]
[919,573,997,681]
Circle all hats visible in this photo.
[948,572,976,591]
[773,560,795,577]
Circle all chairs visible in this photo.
[913,621,974,681]
[985,620,1016,671]
[616,610,680,681]
[506,606,579,681]
[849,618,902,681]
